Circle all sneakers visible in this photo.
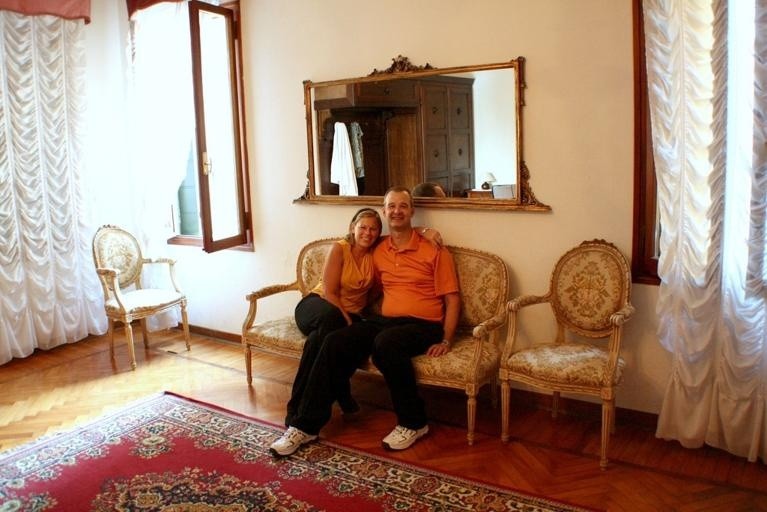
[269,426,318,457]
[382,425,429,450]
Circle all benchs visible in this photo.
[242,238,510,446]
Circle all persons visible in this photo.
[269,185,461,458]
[411,181,445,197]
[284,208,443,429]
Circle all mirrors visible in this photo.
[293,55,550,212]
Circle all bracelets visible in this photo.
[421,227,429,234]
[441,339,451,347]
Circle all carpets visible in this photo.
[1,391,604,511]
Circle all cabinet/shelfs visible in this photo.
[313,74,475,197]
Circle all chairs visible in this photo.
[473,240,636,469]
[94,224,192,369]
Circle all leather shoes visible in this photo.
[337,397,359,414]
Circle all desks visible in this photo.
[465,188,492,198]
